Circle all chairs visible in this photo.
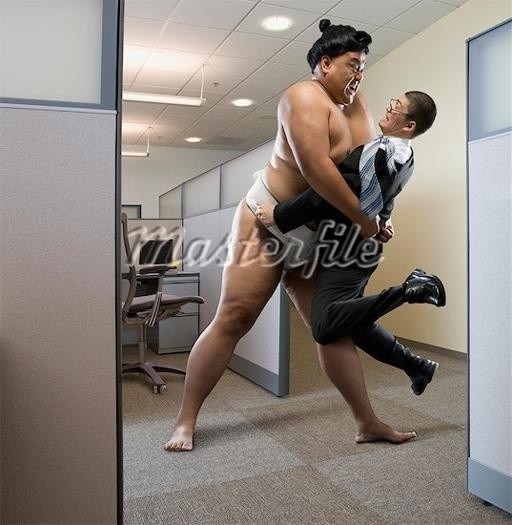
[121,213,204,394]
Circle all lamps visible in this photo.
[122,61,206,109]
[120,127,151,158]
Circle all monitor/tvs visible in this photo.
[137,237,176,266]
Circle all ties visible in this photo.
[359,135,398,221]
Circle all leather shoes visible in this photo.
[402,269,445,306]
[406,356,439,395]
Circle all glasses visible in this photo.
[386,99,407,115]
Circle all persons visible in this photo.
[254,91,446,396]
[164,18,417,452]
